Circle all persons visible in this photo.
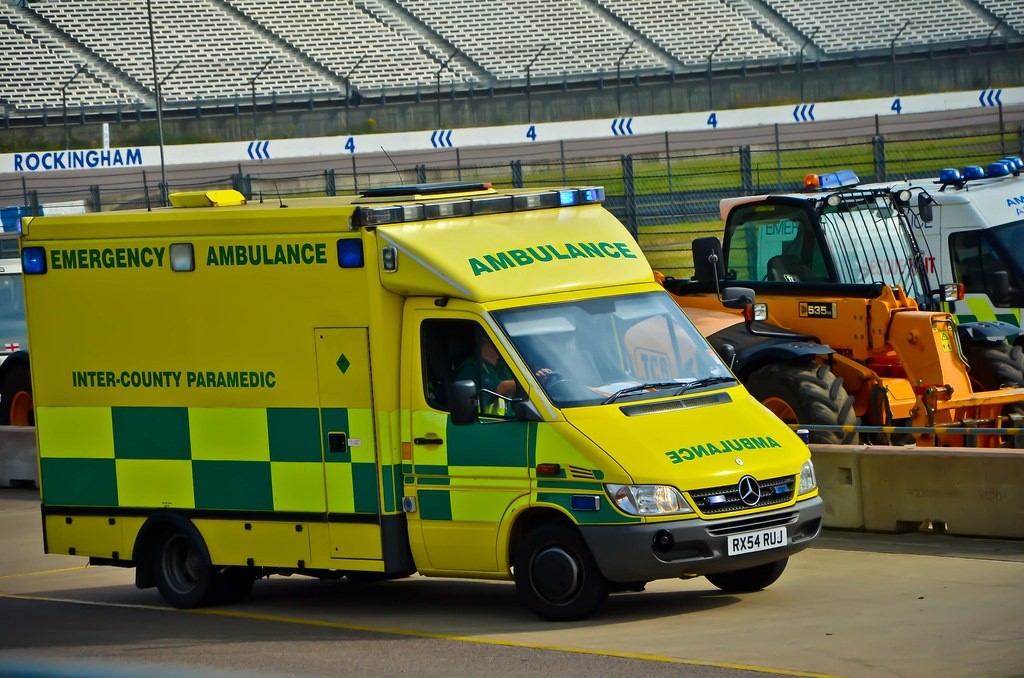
[478,335,550,417]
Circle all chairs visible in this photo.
[546,331,628,386]
[433,340,465,401]
[960,253,1005,294]
[766,254,822,283]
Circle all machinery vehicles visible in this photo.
[659,171,1024,452]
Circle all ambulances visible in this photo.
[717,157,1023,334]
[18,189,825,622]
